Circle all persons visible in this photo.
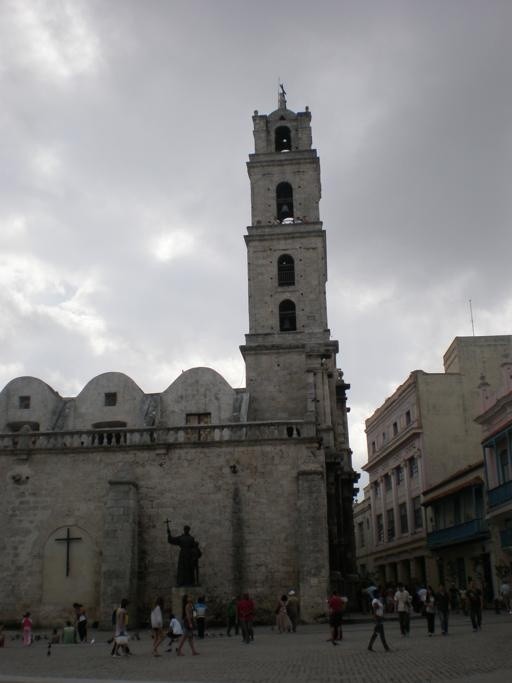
[20,582,511,657]
[168,526,203,586]
